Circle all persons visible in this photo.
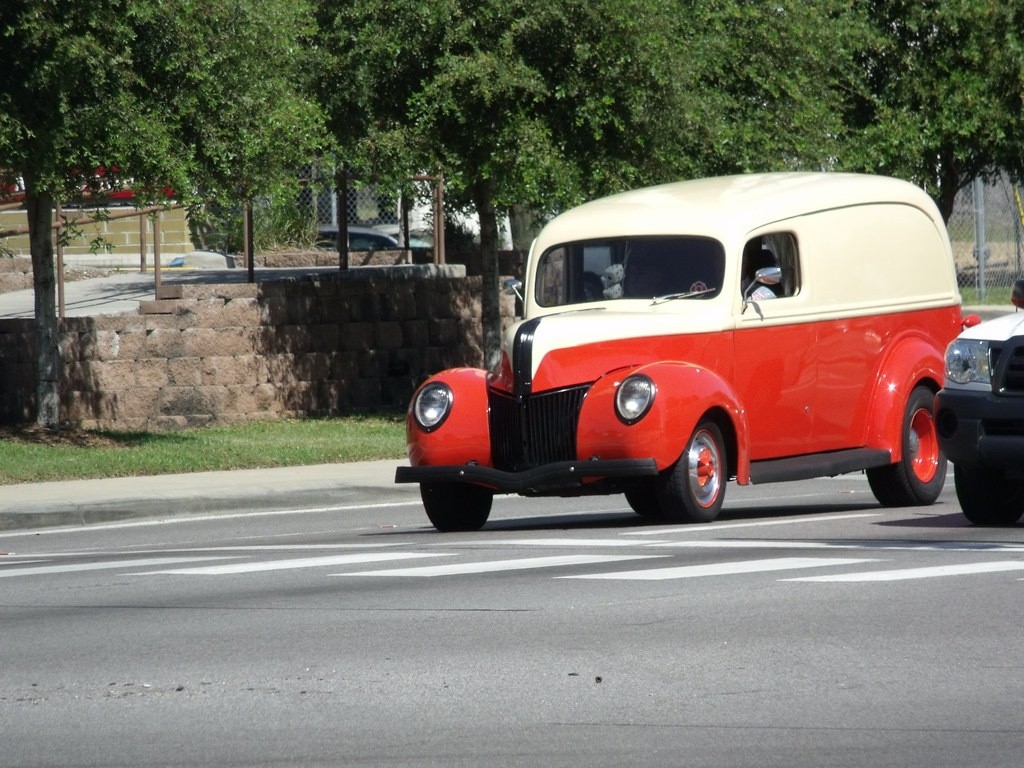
[714,244,776,299]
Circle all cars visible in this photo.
[374,222,435,249]
[934,279,1024,527]
[317,225,398,252]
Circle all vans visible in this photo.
[395,169,965,530]
